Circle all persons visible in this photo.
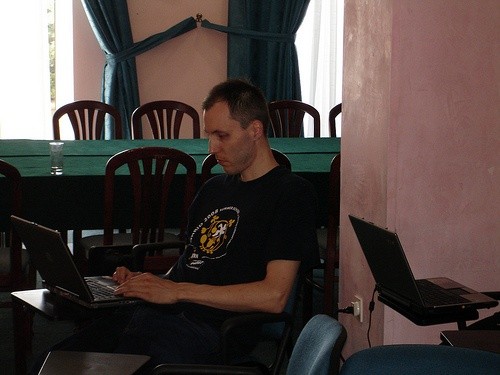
[28,79,323,375]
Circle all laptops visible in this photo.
[348,214,498,313]
[10,216,144,309]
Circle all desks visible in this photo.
[0,138,343,290]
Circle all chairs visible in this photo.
[287,315,500,375]
[0,160,37,366]
[53,99,343,271]
[150,191,320,375]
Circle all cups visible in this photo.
[48,142,64,174]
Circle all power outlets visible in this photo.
[353,295,364,321]
